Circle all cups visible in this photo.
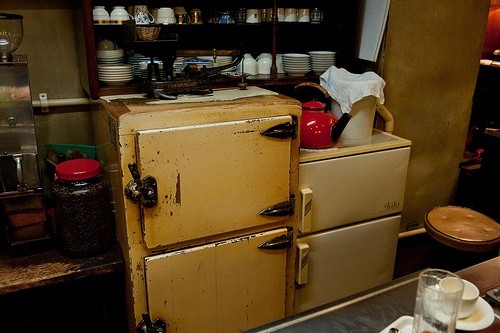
[110,6,134,25]
[92,6,109,25]
[134,5,155,25]
[412,268,465,333]
[153,8,176,25]
[174,7,191,24]
[219,8,323,23]
[438,277,480,320]
[190,9,202,24]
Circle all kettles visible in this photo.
[292,81,352,150]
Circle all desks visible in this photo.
[0,245,124,294]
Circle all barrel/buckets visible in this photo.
[330,95,377,140]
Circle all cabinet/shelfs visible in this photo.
[92,86,302,332]
[74,0,365,97]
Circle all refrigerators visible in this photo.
[294,128,412,316]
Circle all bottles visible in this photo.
[258,53,272,75]
[51,158,116,253]
[243,54,258,75]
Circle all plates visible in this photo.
[380,315,439,333]
[309,51,336,75]
[424,284,495,331]
[281,54,311,76]
[195,56,232,72]
[97,49,190,86]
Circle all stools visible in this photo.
[425,205,500,252]
